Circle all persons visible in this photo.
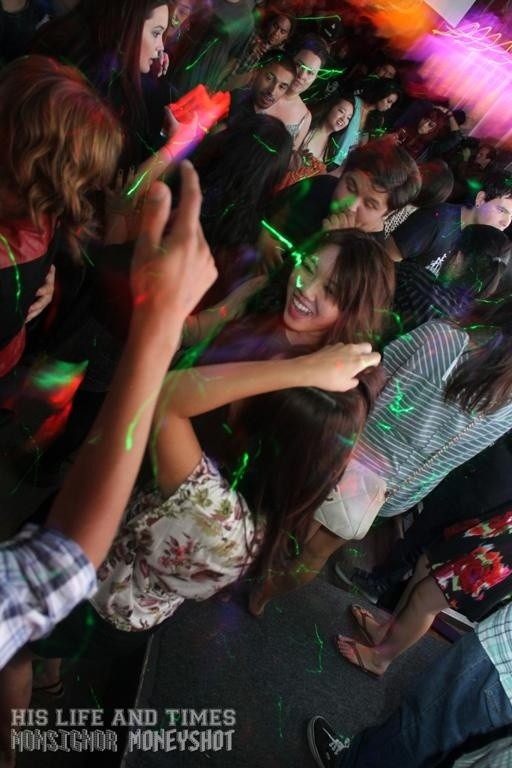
[1,0,511,768]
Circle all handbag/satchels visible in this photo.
[313,457,389,540]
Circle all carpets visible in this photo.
[121,554,453,768]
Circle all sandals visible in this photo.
[32,679,65,698]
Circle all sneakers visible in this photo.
[307,715,350,768]
[335,561,380,604]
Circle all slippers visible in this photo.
[333,604,384,680]
